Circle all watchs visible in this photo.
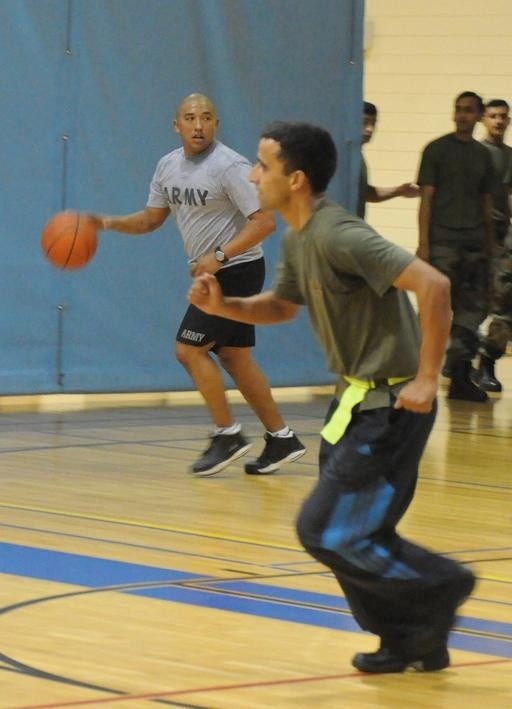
[214,246,229,264]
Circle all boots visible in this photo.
[441,354,502,401]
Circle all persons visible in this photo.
[474,98,512,392]
[88,94,308,475]
[358,100,421,224]
[414,90,497,402]
[185,121,476,675]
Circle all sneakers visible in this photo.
[402,564,475,659]
[352,645,450,674]
[244,428,308,475]
[193,423,253,476]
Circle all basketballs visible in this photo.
[41,211,96,268]
